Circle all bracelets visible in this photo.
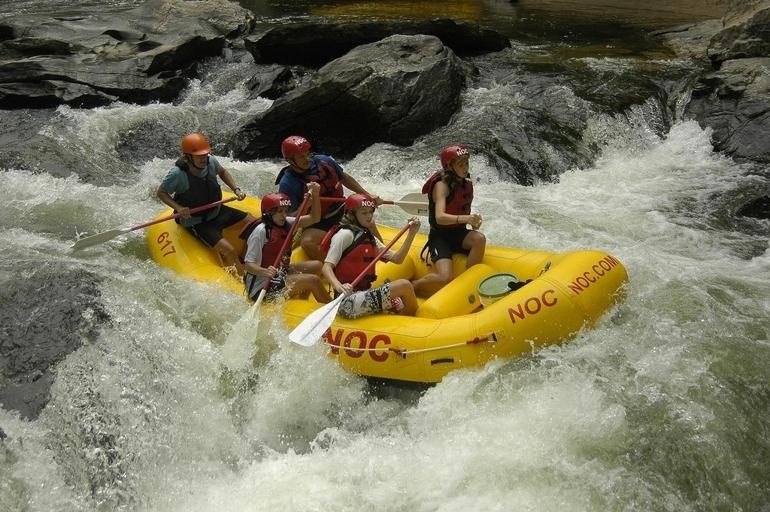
[457,215,460,224]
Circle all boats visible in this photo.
[146,189,632,390]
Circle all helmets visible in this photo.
[441,144,469,167]
[343,193,376,209]
[261,192,291,212]
[182,134,210,156]
[282,136,311,157]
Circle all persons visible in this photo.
[243,181,333,304]
[157,133,256,276]
[275,136,383,265]
[411,144,486,298]
[322,193,418,320]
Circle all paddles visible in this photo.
[70,195,238,249]
[221,187,313,369]
[319,192,430,217]
[289,220,413,347]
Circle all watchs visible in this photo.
[234,185,241,194]
